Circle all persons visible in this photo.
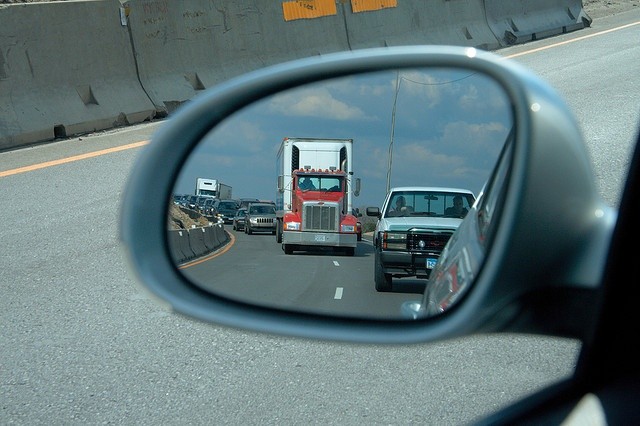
[389,196,414,215]
[446,195,469,217]
[300,177,317,189]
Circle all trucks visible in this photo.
[195,176,233,199]
[276,137,360,255]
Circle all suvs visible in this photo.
[367,186,476,291]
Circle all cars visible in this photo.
[402,124,516,321]
[173,194,276,234]
[352,207,362,241]
